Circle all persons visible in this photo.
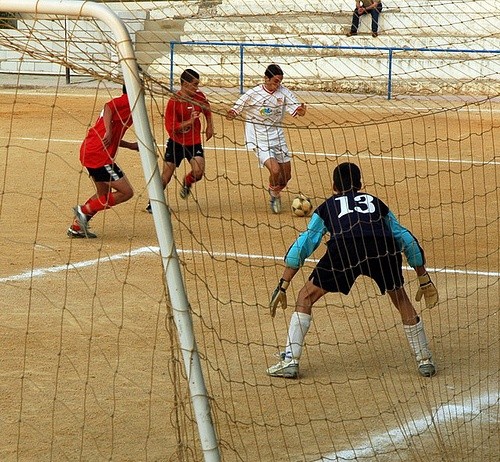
[70,79,146,238]
[147,67,214,215]
[349,0,382,38]
[226,65,306,216]
[266,162,440,377]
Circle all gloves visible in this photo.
[415,274,438,310]
[269,279,287,317]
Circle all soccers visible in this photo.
[291,195,312,217]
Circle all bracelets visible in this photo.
[365,8,367,11]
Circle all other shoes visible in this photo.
[372,32,376,38]
[347,31,357,36]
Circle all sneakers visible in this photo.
[72,205,89,236]
[180,181,191,199]
[147,203,152,213]
[269,188,281,214]
[68,227,96,239]
[418,364,434,376]
[266,352,299,378]
